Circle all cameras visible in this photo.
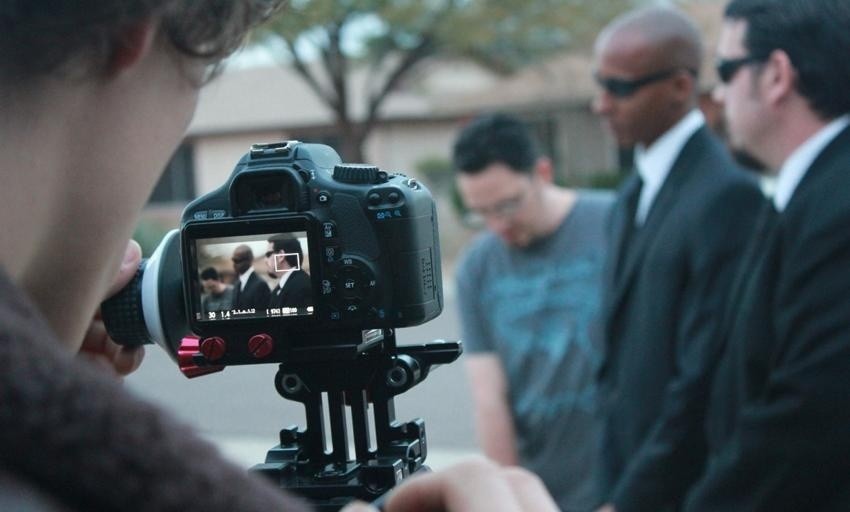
[101,139,443,379]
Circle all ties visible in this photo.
[702,194,776,455]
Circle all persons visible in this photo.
[684,0,849,512]
[199,235,312,317]
[0,0,567,512]
[585,0,760,512]
[455,109,617,512]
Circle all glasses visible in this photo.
[715,53,767,82]
[231,257,249,264]
[592,68,699,99]
[265,250,278,257]
